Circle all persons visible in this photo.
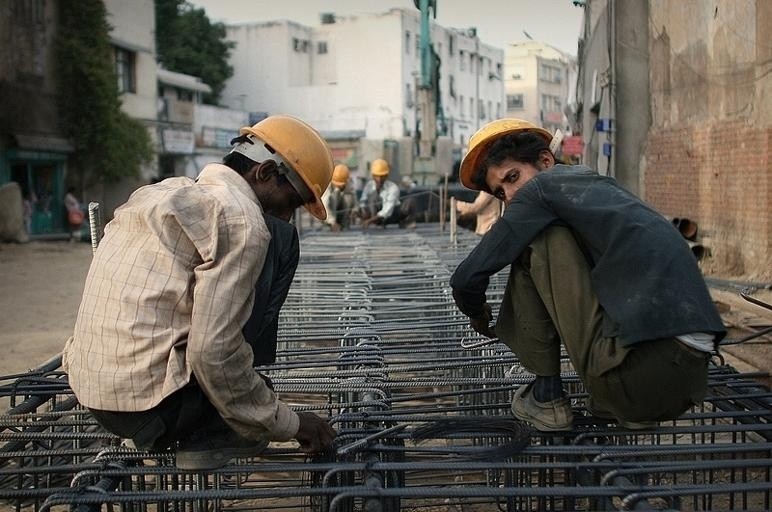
[449,118,729,433]
[356,158,416,230]
[64,186,84,244]
[23,192,33,235]
[320,163,359,231]
[65,113,339,470]
[456,190,501,236]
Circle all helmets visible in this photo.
[370,159,390,177]
[239,114,336,221]
[331,164,350,187]
[458,117,554,191]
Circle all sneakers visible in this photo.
[584,396,661,430]
[175,425,271,470]
[510,383,576,432]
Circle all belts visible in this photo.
[658,335,712,375]
